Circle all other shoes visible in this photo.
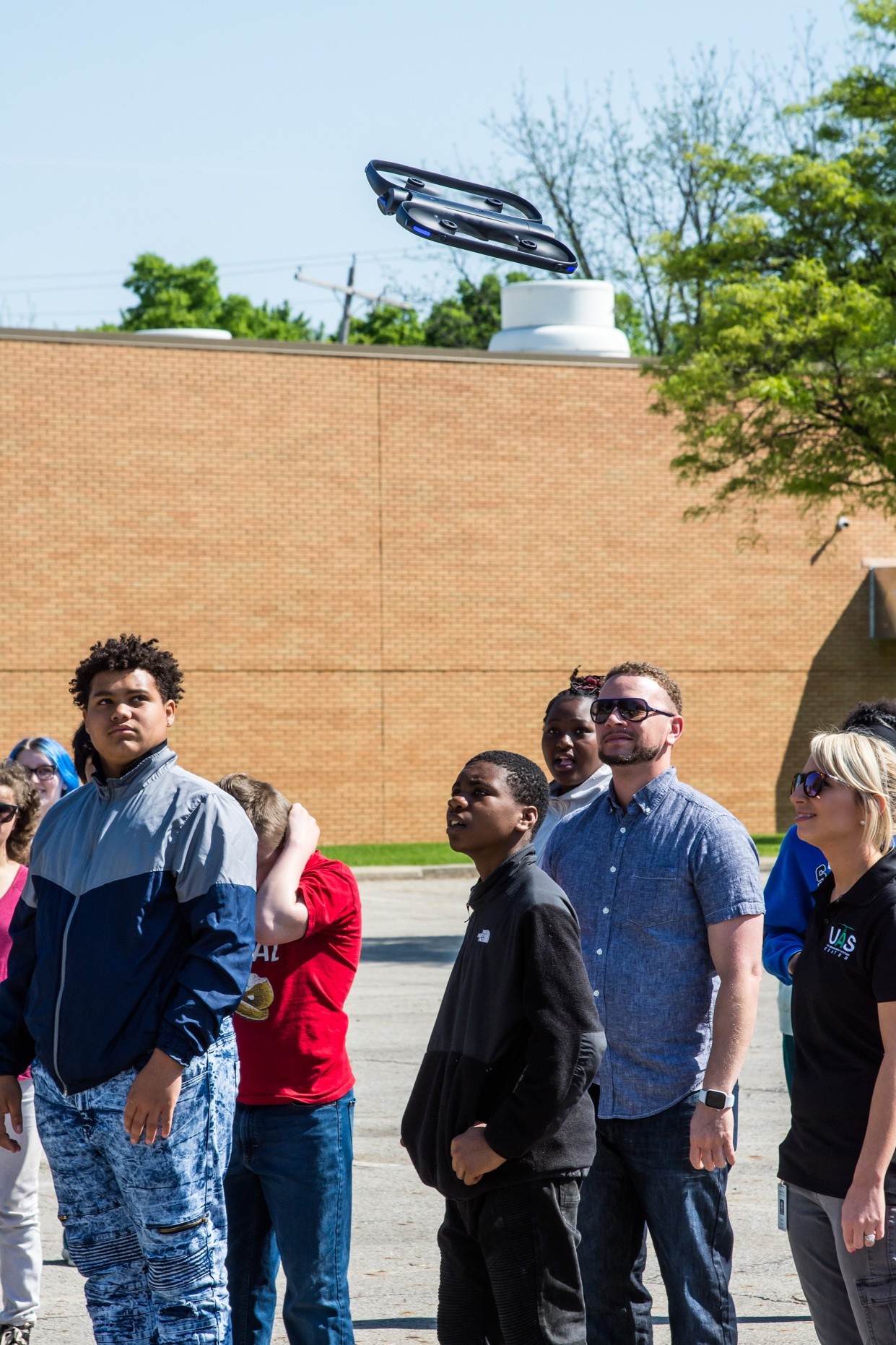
[62,1249,77,1267]
[0,1322,33,1345]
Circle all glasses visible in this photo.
[22,765,57,781]
[0,804,19,823]
[590,697,675,724]
[791,770,846,798]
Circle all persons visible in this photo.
[400,750,608,1345]
[0,720,96,1345]
[537,664,766,1345]
[0,631,257,1345]
[763,698,896,1345]
[533,688,612,869]
[209,771,363,1345]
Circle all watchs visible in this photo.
[699,1089,735,1112]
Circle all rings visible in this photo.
[863,1234,876,1243]
